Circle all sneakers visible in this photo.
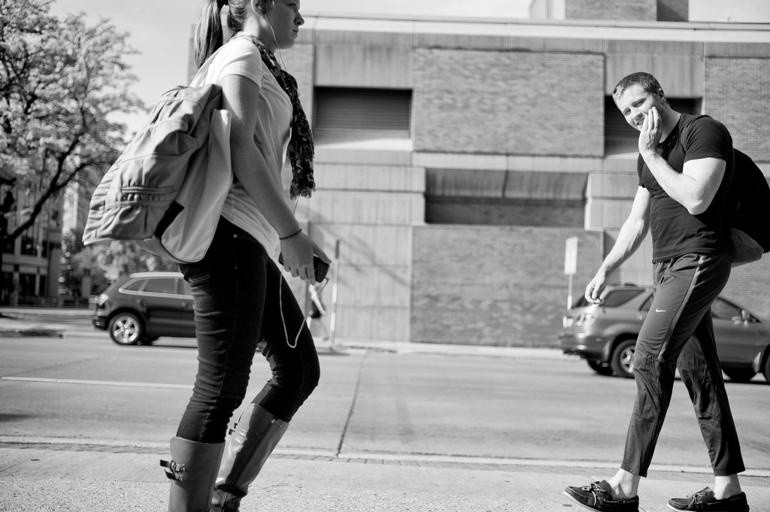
[667,486,749,512]
[565,482,639,512]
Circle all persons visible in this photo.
[154,0,333,512]
[562,70,752,512]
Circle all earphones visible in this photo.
[258,3,267,15]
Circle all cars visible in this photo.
[558,282,770,383]
[91,271,196,344]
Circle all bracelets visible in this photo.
[279,227,303,241]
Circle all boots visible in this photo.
[210,401,288,512]
[161,436,225,512]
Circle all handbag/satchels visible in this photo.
[135,109,233,264]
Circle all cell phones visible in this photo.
[278,250,329,284]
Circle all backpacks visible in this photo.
[680,115,769,265]
